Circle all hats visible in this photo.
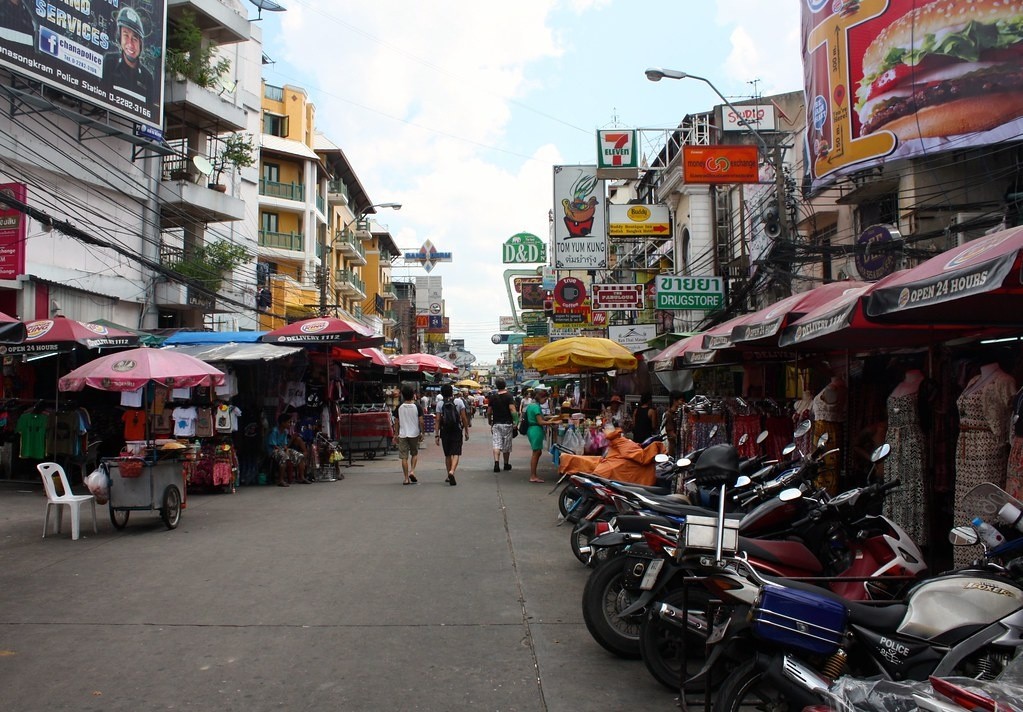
[610,396,623,404]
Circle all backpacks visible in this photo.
[441,397,462,433]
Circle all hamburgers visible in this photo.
[854,0,1023,139]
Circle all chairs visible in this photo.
[549,442,575,495]
[37,462,98,540]
[68,440,103,486]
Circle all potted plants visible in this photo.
[171,167,193,181]
[208,130,257,192]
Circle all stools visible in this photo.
[269,460,300,485]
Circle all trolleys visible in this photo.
[102,441,193,530]
[341,413,393,460]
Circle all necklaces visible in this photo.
[894,381,917,415]
[960,367,1000,416]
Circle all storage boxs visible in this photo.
[679,515,740,556]
[318,467,337,482]
[748,585,845,653]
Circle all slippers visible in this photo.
[408,474,417,482]
[299,477,311,485]
[278,481,289,487]
[403,479,410,485]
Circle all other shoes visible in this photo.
[504,463,511,470]
[529,476,544,483]
[445,474,456,485]
[494,466,500,472]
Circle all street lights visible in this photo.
[407,307,441,353]
[645,66,793,296]
[320,203,402,318]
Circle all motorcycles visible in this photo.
[549,408,1023,712]
[482,404,488,418]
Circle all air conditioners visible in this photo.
[947,213,1006,248]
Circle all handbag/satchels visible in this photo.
[329,450,345,464]
[84,463,110,505]
[513,429,518,438]
[520,410,528,435]
[118,460,145,479]
[563,428,610,455]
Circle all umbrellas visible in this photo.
[0,312,399,372]
[454,379,481,389]
[394,350,459,381]
[641,224,1023,398]
[59,343,226,449]
[262,315,385,396]
[523,337,638,400]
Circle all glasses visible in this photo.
[612,401,619,404]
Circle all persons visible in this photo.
[883,370,937,547]
[954,363,1018,572]
[435,383,469,486]
[392,385,425,485]
[265,413,319,487]
[413,386,586,436]
[102,7,154,106]
[791,390,815,467]
[525,390,563,482]
[812,377,848,498]
[487,376,519,472]
[601,390,686,450]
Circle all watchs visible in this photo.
[435,436,439,438]
